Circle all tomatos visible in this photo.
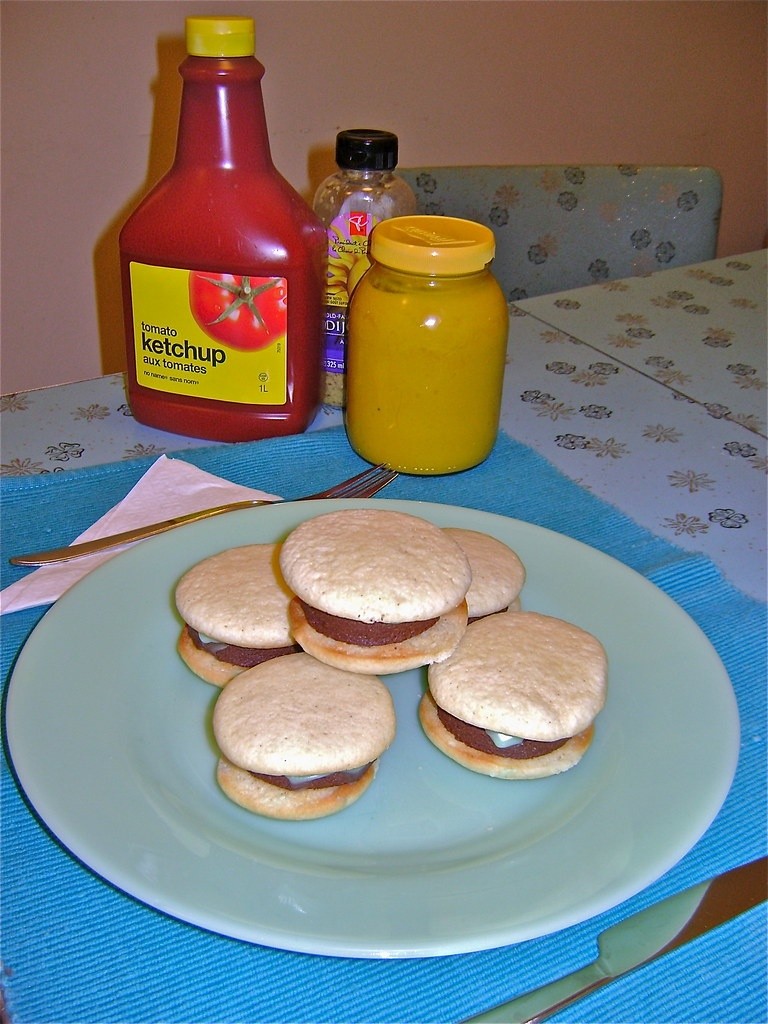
[187,271,287,353]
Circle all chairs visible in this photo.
[392,164,723,302]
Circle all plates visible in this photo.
[7,499,739,959]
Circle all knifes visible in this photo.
[451,879,715,1024]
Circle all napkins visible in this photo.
[0,454,284,615]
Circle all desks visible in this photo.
[0,247,768,1024]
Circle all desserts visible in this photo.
[174,509,606,821]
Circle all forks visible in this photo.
[8,462,398,566]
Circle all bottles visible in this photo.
[349,215,509,478]
[119,16,325,442]
[316,130,415,407]
